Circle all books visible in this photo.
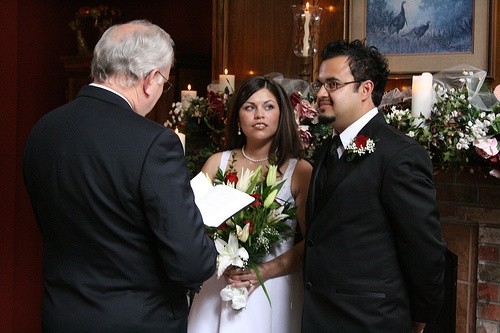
[190,171,256,229]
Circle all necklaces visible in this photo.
[241,145,269,162]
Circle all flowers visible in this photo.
[163,66,500,180]
[205,151,298,311]
[345,134,377,164]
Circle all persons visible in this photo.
[300,39,455,333]
[187,75,313,333]
[23,19,215,333]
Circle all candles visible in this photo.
[174,127,185,157]
[412,70,433,128]
[180,83,197,117]
[219,68,235,95]
[302,1,311,57]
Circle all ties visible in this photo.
[313,136,342,204]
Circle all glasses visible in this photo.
[144,68,173,92]
[312,80,365,92]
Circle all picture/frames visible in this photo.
[342,0,497,83]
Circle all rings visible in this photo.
[249,280,252,289]
